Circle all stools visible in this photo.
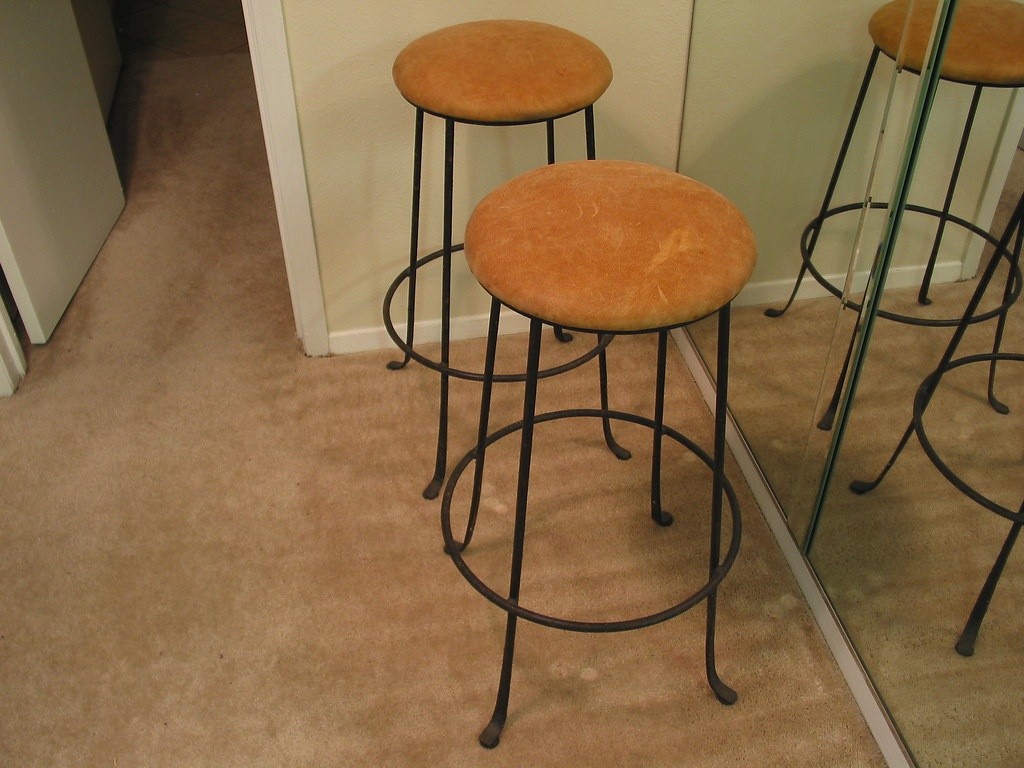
[848,196,1024,659]
[441,161,754,749]
[382,18,636,462]
[763,0,1024,430]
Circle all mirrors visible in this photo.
[665,0,1024,768]
